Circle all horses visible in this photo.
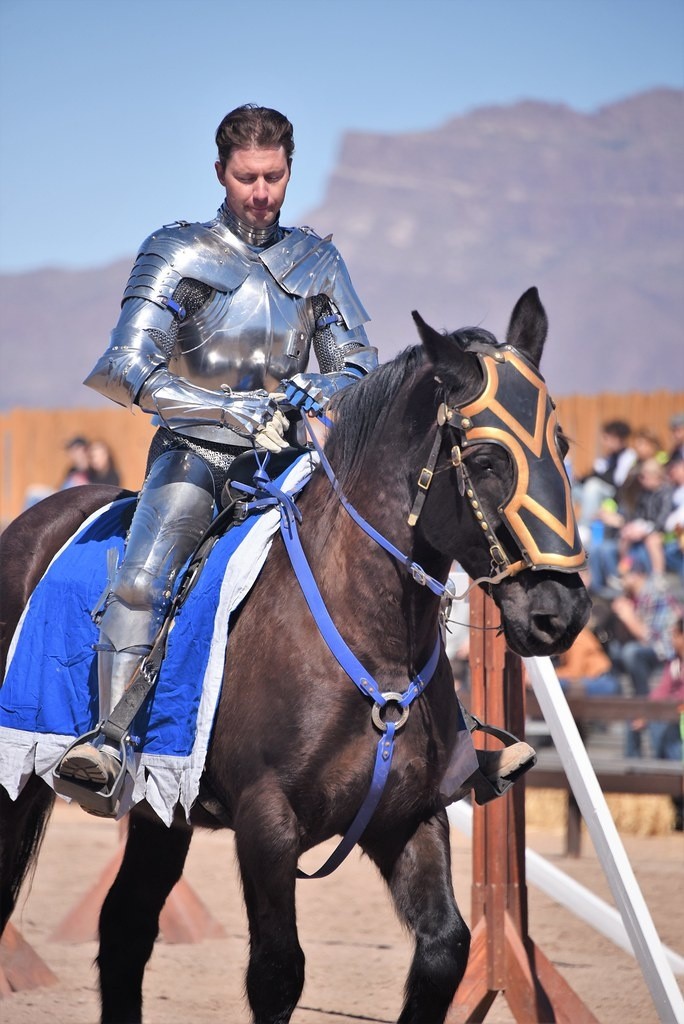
[0,286,593,1024]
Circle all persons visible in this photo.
[573,411,684,834]
[86,441,121,486]
[62,437,97,491]
[61,103,542,818]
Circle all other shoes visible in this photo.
[59,744,121,818]
[440,741,537,807]
